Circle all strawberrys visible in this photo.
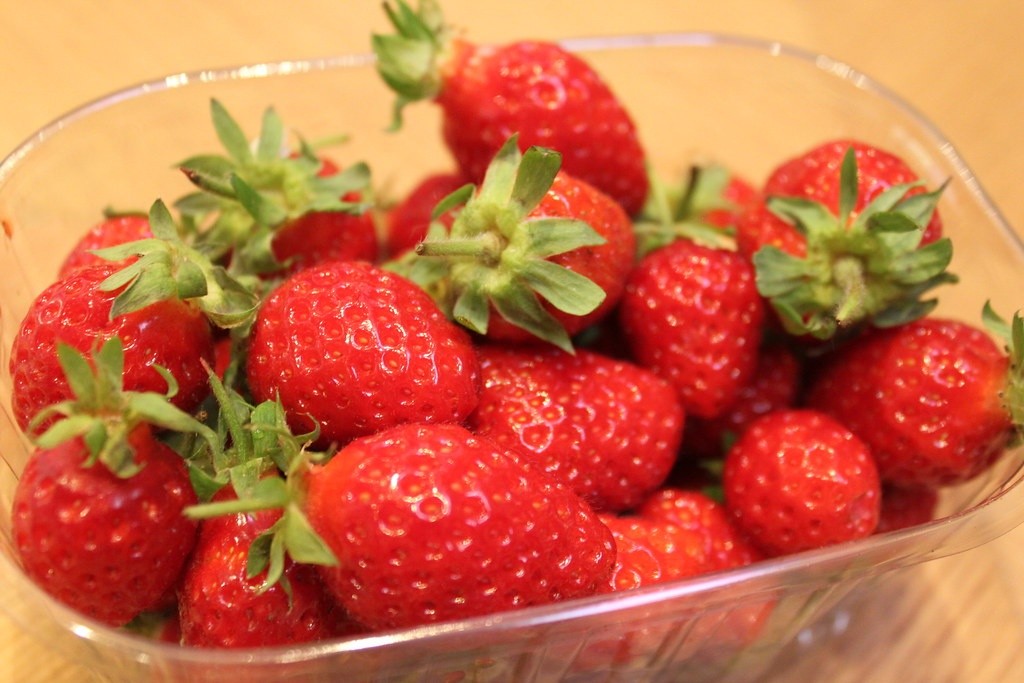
[10,2,1024,680]
